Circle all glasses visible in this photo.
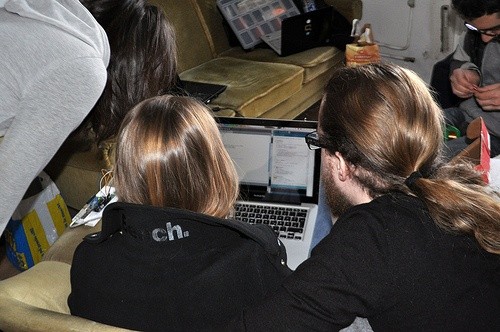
[464,20,500,37]
[305,131,335,155]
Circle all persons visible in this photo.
[220,60,500,332]
[0,0,179,238]
[67,95,293,332]
[430,0,500,166]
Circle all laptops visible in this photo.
[211,116,322,271]
[170,80,227,106]
[261,5,334,56]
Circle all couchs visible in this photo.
[54,0,362,212]
[0,175,146,331]
